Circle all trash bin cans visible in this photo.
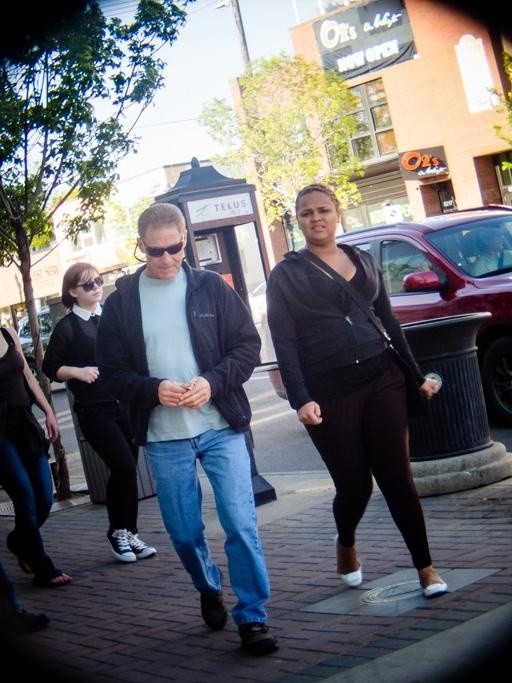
[382,312,493,462]
[63,383,157,506]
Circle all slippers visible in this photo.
[31,568,71,588]
[6,530,33,574]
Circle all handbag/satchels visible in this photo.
[387,349,425,419]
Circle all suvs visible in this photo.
[259,203,512,433]
[16,306,61,389]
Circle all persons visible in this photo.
[0,563,53,635]
[0,322,75,591]
[95,199,281,652]
[39,260,157,565]
[264,184,448,597]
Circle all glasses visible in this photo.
[139,233,185,257]
[75,276,104,291]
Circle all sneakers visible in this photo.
[106,524,137,562]
[238,622,277,650]
[200,567,228,631]
[0,608,50,635]
[128,530,157,559]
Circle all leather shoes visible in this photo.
[419,568,448,599]
[334,532,363,587]
